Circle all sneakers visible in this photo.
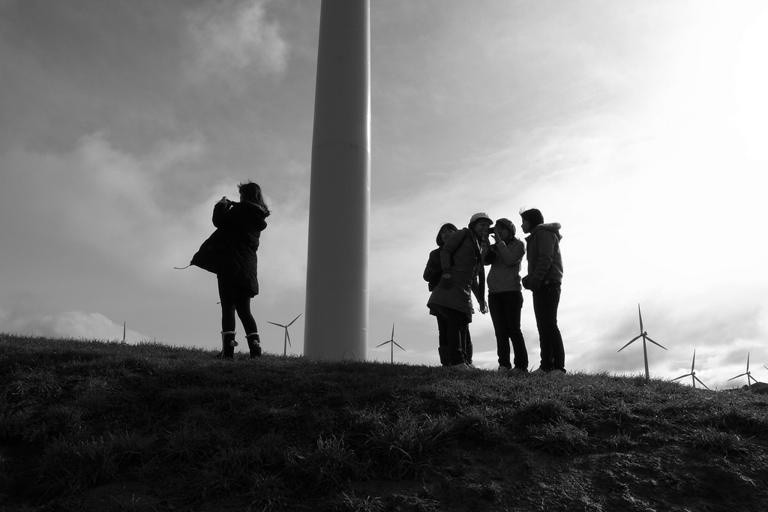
[549,369,565,376]
[442,362,469,370]
[513,367,527,373]
[497,364,510,370]
[459,362,475,369]
[532,367,548,375]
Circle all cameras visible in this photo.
[489,228,494,234]
[226,199,232,208]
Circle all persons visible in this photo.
[519,207,570,373]
[427,213,492,369]
[189,177,270,359]
[480,218,529,376]
[423,221,488,369]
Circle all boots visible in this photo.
[244,332,263,360]
[217,332,237,361]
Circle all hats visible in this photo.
[468,213,493,227]
